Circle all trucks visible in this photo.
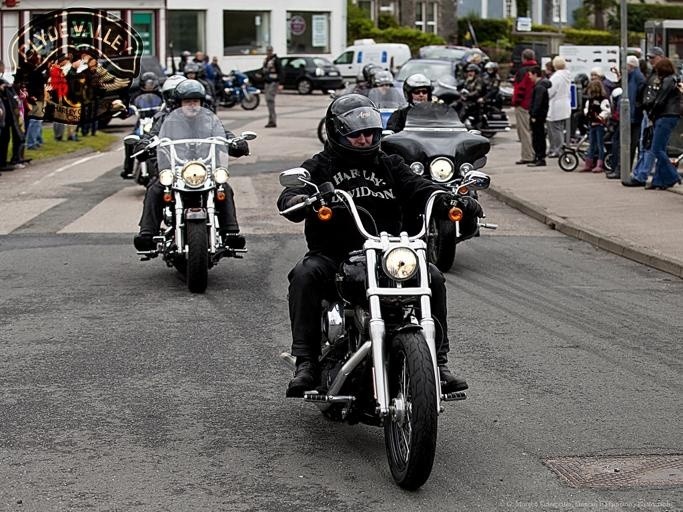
[541,43,642,76]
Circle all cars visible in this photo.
[242,57,345,94]
[395,43,512,105]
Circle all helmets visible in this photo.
[362,62,393,90]
[174,79,206,117]
[326,93,382,162]
[403,75,434,103]
[467,62,500,77]
[140,72,158,91]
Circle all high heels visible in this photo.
[644,175,682,192]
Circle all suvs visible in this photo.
[0,55,167,129]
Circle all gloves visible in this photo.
[284,194,310,217]
[463,195,484,216]
[135,139,151,161]
[234,138,249,156]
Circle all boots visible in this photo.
[577,157,606,174]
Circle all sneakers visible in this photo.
[264,121,278,129]
[2,129,100,172]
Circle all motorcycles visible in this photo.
[111,66,260,293]
[277,166,495,491]
[365,83,510,274]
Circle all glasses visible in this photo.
[413,89,430,95]
[346,130,374,138]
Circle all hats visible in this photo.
[646,46,664,57]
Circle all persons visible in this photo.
[258,45,285,128]
[133,79,246,258]
[274,92,485,397]
[0,49,230,184]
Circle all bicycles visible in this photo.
[557,125,617,172]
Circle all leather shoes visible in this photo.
[439,365,468,392]
[223,230,248,253]
[621,178,646,188]
[286,360,318,397]
[134,233,157,258]
[514,157,548,167]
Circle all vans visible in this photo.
[333,38,411,78]
